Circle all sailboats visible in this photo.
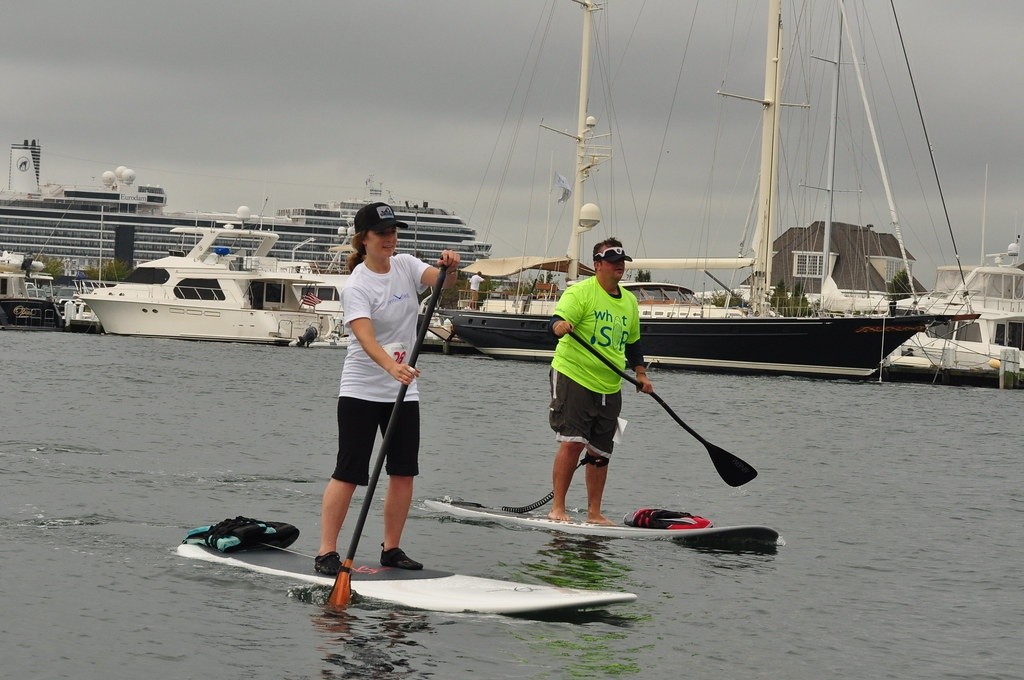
[435,1,982,379]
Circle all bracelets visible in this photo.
[445,270,456,275]
[636,371,645,376]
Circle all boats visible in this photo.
[0,252,119,333]
[76,220,339,348]
[880,237,1024,388]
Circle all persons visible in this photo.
[469,271,484,311]
[21,251,34,278]
[547,236,652,528]
[313,202,461,574]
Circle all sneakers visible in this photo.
[380,549,424,569]
[315,552,342,576]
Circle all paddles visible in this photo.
[567,326,758,487]
[326,254,447,604]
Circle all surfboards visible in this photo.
[425,498,779,543]
[176,539,637,615]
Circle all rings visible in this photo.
[408,367,415,373]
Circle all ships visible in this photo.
[0,138,493,283]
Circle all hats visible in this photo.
[354,202,408,233]
[593,247,632,264]
